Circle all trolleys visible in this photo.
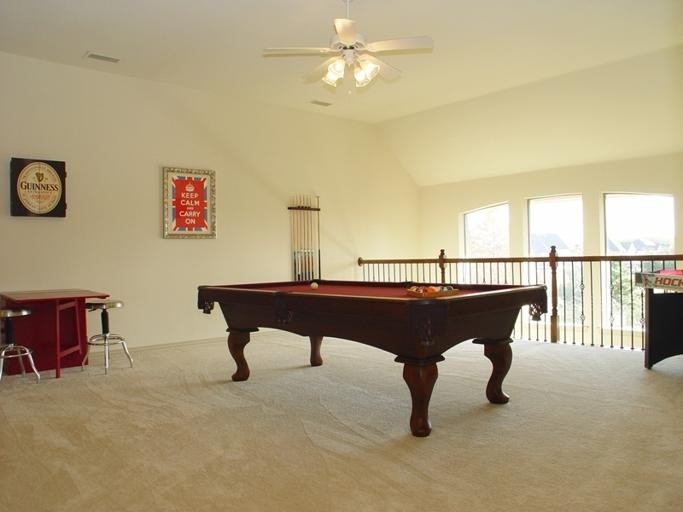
[261,0,433,84]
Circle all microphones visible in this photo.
[0,308,44,383]
[80,300,134,375]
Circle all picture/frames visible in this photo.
[1,289,110,378]
[195,277,547,438]
[634,268,683,371]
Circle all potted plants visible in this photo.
[161,166,217,240]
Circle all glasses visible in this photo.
[319,52,380,89]
